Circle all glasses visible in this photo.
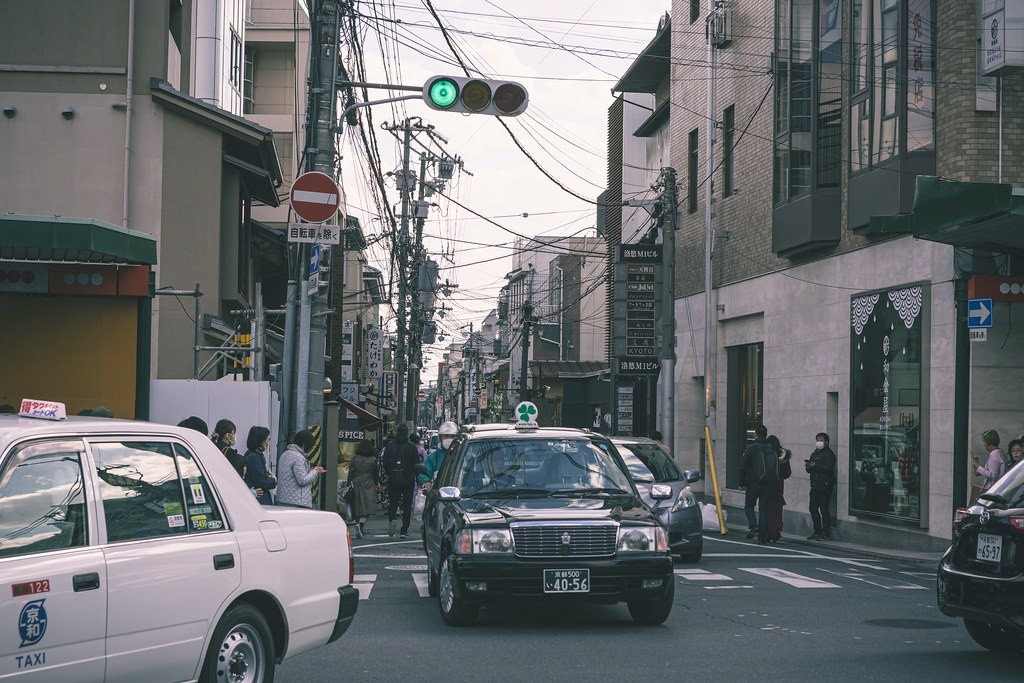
[266,438,271,442]
[1011,449,1022,454]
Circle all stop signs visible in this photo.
[289,171,341,224]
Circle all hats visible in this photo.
[90,406,114,418]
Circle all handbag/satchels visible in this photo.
[343,486,355,522]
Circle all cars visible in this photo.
[417,399,593,497]
[597,433,705,564]
[0,392,362,683]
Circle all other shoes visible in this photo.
[747,528,759,539]
[388,519,397,537]
[817,534,830,541]
[355,522,365,539]
[400,532,410,538]
[806,530,822,540]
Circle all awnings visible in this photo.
[221,153,281,208]
[529,360,610,378]
[913,203,1024,259]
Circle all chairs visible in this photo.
[0,464,78,557]
[540,451,590,488]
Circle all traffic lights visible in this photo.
[421,74,529,117]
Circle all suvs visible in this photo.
[935,458,1024,665]
[420,400,675,629]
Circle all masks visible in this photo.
[227,433,236,446]
[816,440,829,450]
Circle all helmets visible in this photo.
[438,422,459,442]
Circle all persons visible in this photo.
[241,426,278,505]
[737,424,781,544]
[465,446,516,490]
[647,431,672,478]
[973,429,1006,499]
[418,422,459,489]
[1005,428,1024,474]
[211,419,264,500]
[371,426,432,515]
[383,423,419,537]
[178,416,209,437]
[77,406,114,419]
[805,433,837,542]
[766,435,792,542]
[276,430,328,509]
[347,439,380,539]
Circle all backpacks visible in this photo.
[754,443,780,483]
[389,440,412,488]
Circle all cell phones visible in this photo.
[804,459,811,463]
